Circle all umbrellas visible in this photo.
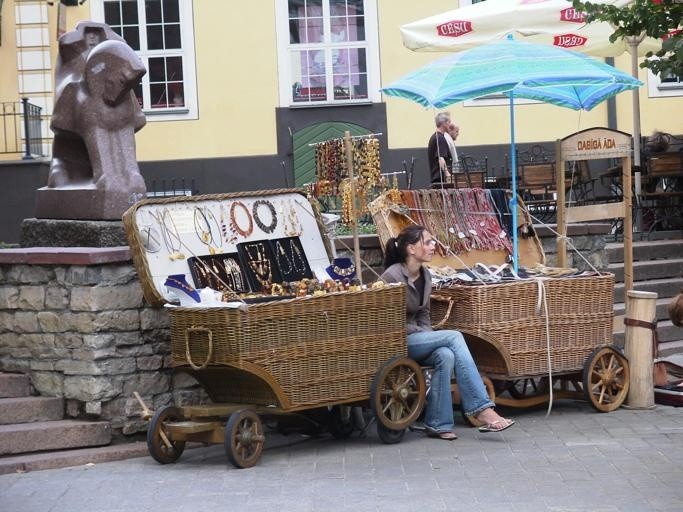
[377,31,647,285]
[401,0,669,60]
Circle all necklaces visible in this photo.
[165,195,313,292]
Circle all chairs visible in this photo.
[437,128,682,243]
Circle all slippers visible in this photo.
[478,417,515,432]
[428,433,456,439]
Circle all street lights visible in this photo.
[605,1,661,232]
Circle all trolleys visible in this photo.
[118,185,437,470]
[362,185,630,425]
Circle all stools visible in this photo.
[400,365,436,431]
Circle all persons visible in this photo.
[443,121,460,173]
[427,111,452,184]
[375,222,515,441]
